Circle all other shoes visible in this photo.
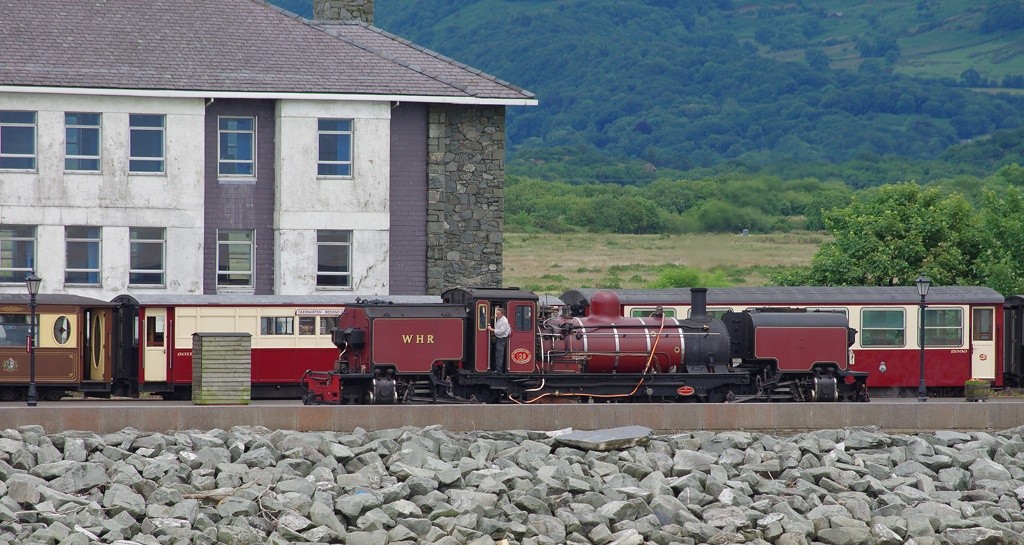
[491,371,503,375]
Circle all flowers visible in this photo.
[969,378,985,383]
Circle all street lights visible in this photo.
[25,268,44,406]
[914,272,929,402]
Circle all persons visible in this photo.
[487,308,511,375]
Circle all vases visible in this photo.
[965,382,991,402]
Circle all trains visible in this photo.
[0,287,1024,404]
